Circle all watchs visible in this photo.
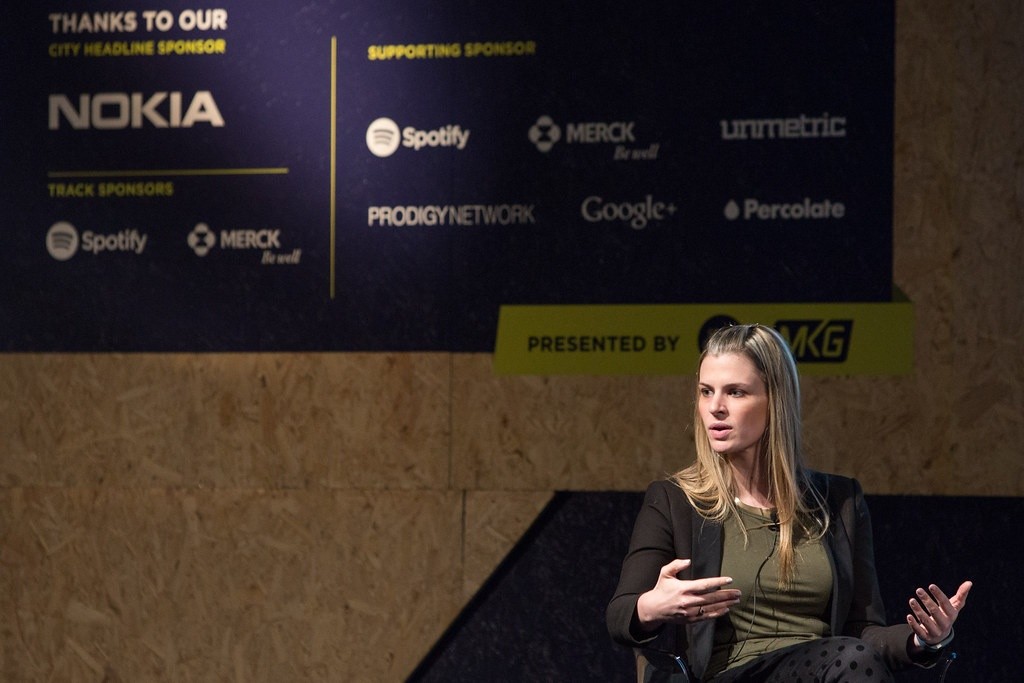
[916,625,954,653]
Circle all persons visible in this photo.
[605,321,974,682]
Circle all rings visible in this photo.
[698,605,705,616]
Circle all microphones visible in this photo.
[769,512,780,531]
